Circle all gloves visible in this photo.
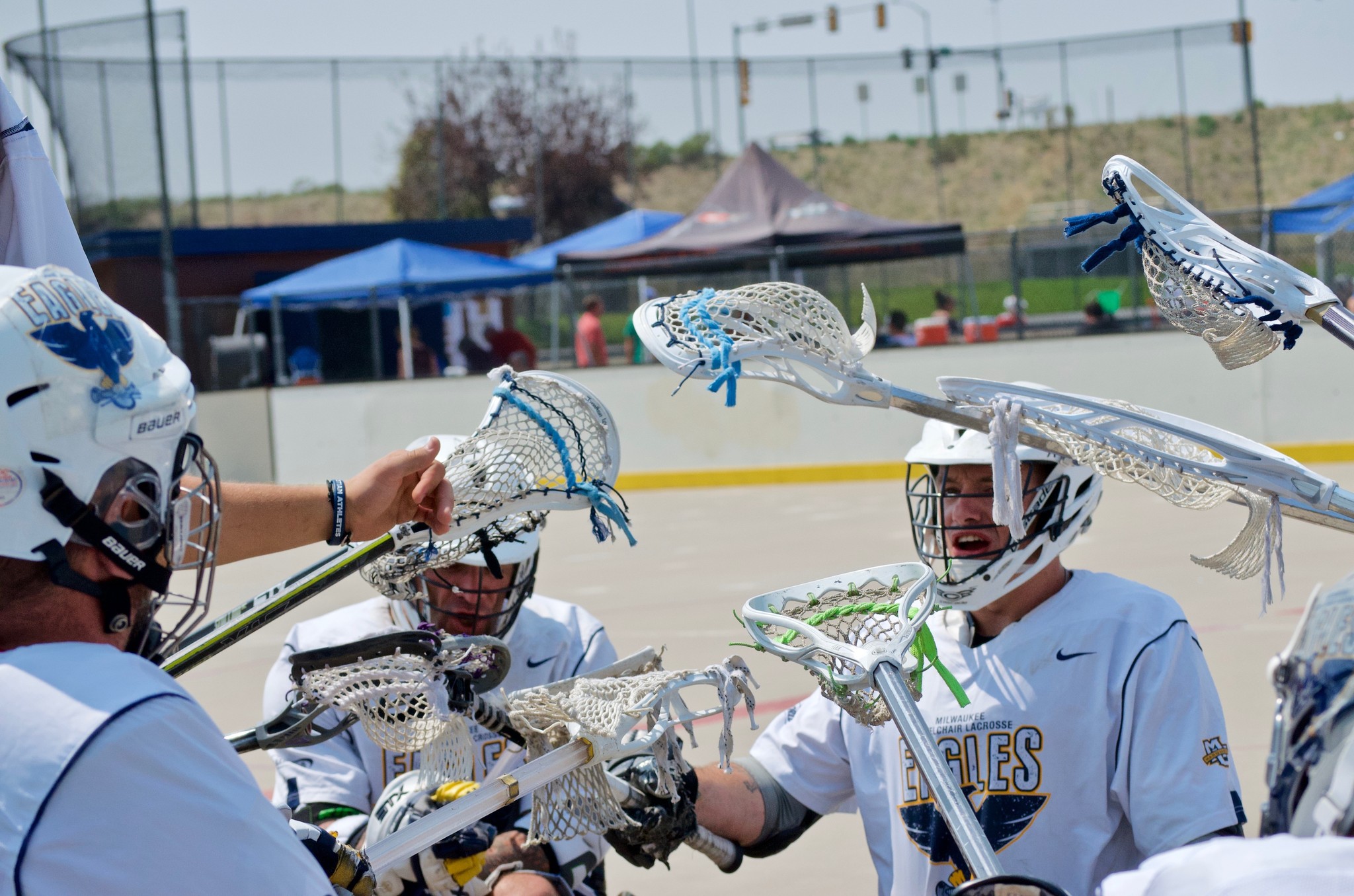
[600,757,698,869]
[361,768,495,894]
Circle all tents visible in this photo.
[511,208,687,363]
[549,140,971,370]
[242,237,555,386]
[1262,172,1354,261]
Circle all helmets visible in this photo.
[903,381,1102,613]
[393,434,540,632]
[1,263,199,561]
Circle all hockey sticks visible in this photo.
[1101,154,1354,370]
[741,562,1009,880]
[159,486,552,661]
[359,654,748,878]
[935,375,1354,519]
[298,654,744,874]
[632,281,1354,534]
[160,370,621,679]
[222,631,511,755]
[477,646,658,790]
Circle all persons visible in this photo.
[1260,576,1354,840]
[931,290,961,334]
[874,309,915,348]
[622,286,663,365]
[484,326,538,373]
[639,403,1246,896]
[395,323,442,379]
[260,433,618,896]
[575,295,609,368]
[1,262,453,896]
[1076,300,1128,338]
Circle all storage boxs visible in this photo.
[913,315,949,348]
[961,313,999,344]
